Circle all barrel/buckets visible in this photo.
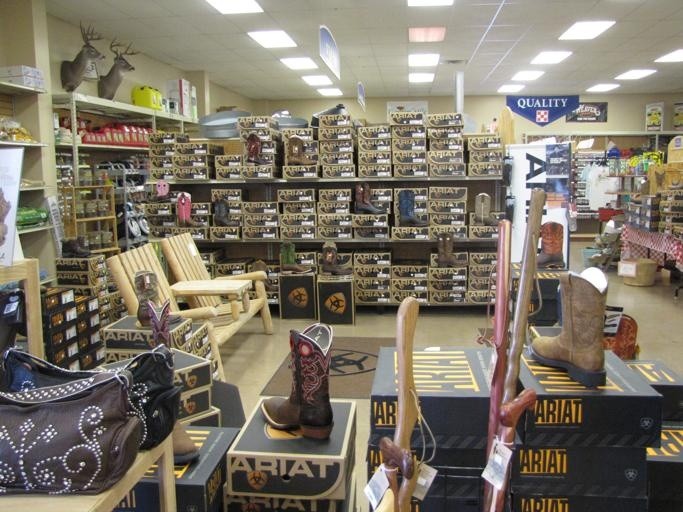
[620,258,657,286]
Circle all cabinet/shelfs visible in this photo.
[0,79,56,287]
[56,183,122,258]
[50,93,200,246]
[603,175,643,207]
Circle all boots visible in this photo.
[258,321,335,442]
[320,240,355,276]
[474,191,492,227]
[176,190,194,227]
[536,221,566,269]
[250,256,279,294]
[397,189,431,228]
[214,194,240,227]
[353,181,387,215]
[436,232,471,269]
[531,265,610,390]
[286,134,318,166]
[146,298,174,356]
[246,133,272,166]
[132,268,184,328]
[603,312,639,362]
[279,240,313,275]
[147,180,172,204]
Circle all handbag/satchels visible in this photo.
[0,341,185,454]
[0,366,144,499]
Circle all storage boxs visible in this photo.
[624,185,682,244]
[146,186,502,239]
[0,76,46,89]
[149,112,501,175]
[0,65,44,79]
[190,86,198,119]
[1,252,681,511]
[163,77,190,118]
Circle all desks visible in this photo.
[618,222,682,301]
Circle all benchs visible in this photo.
[103,232,276,385]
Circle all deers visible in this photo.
[98,37,141,100]
[61,21,105,92]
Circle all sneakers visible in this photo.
[60,236,94,259]
